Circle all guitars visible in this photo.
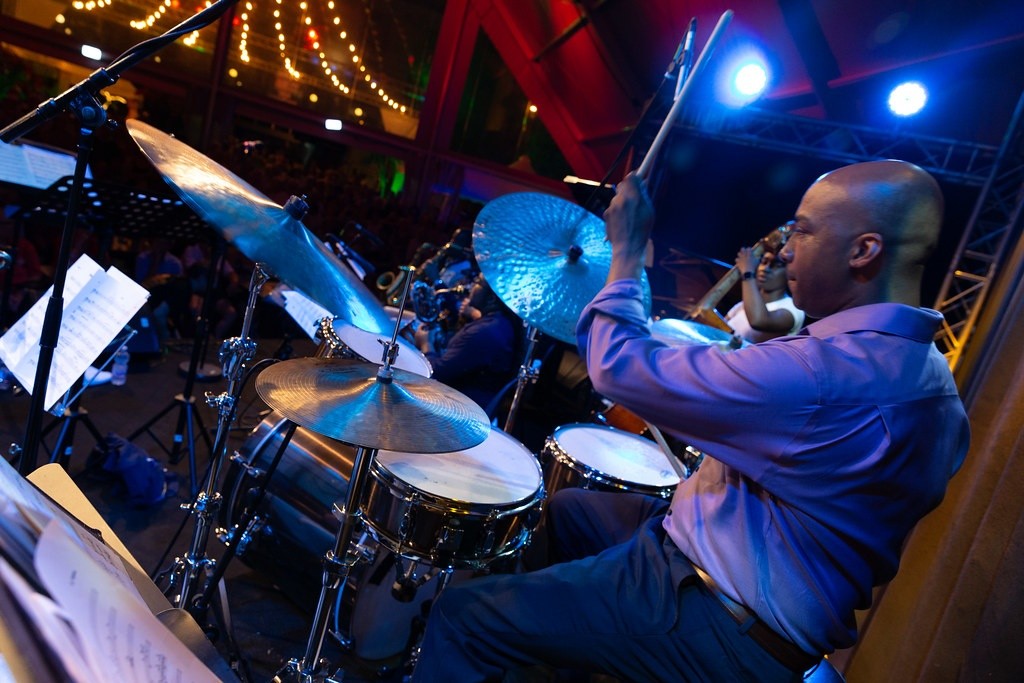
[684,228,785,333]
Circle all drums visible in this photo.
[356,425,546,570]
[311,314,434,383]
[223,411,362,585]
[546,422,690,497]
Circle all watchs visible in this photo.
[740,270,755,281]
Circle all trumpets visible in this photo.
[377,228,486,323]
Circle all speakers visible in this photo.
[95,316,159,367]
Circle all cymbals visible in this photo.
[255,358,490,455]
[470,192,652,347]
[650,318,758,350]
[125,118,395,338]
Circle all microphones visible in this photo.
[668,18,698,126]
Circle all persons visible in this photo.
[4,73,408,348]
[415,271,514,409]
[722,243,806,344]
[410,159,969,683]
